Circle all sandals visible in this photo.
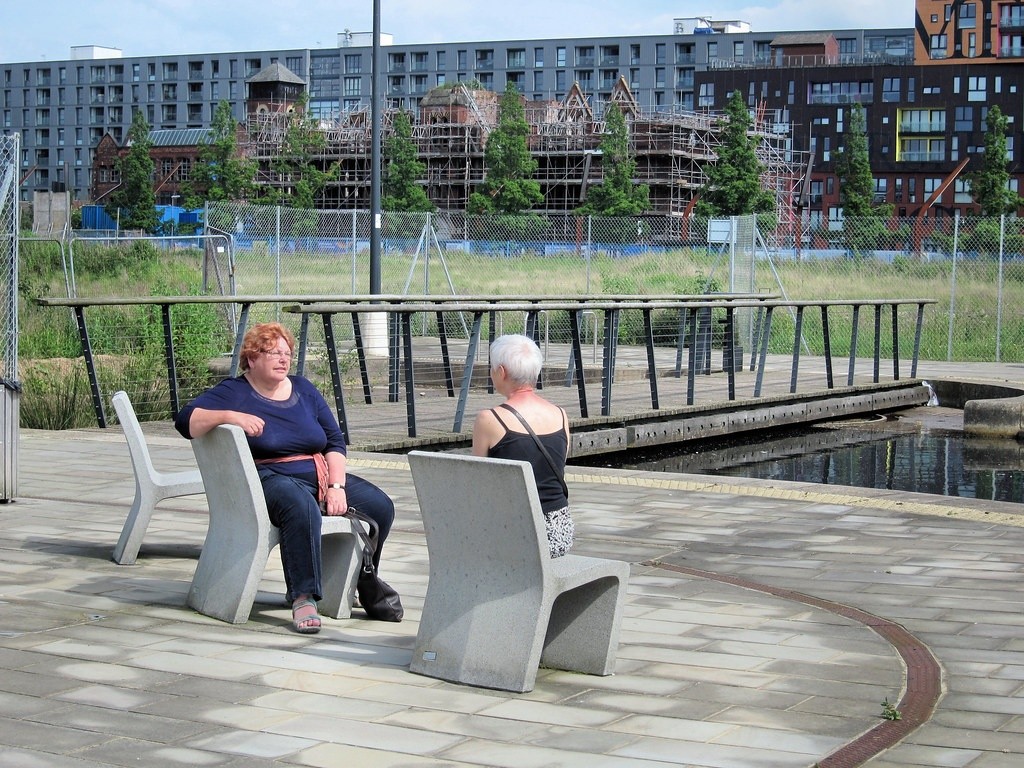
[353,593,364,607]
[291,597,322,634]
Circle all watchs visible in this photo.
[328,483,345,489]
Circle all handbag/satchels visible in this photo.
[357,564,404,623]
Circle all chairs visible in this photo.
[112,391,205,565]
[187,423,372,625]
[406,449,630,693]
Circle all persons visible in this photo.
[175,324,395,633]
[472,334,573,557]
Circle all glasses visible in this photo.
[259,349,293,360]
